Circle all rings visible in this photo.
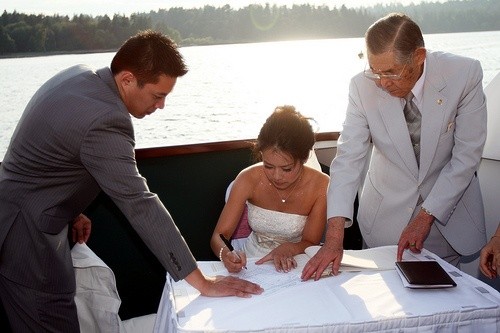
[410,244,415,246]
[288,259,292,262]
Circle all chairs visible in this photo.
[225,181,264,250]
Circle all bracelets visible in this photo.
[491,235,500,240]
[219,245,227,261]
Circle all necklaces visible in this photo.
[268,179,298,203]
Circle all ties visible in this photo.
[402,91,422,169]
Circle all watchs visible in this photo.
[422,208,432,216]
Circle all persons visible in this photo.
[210,106,331,272]
[479,223,500,279]
[300,15,488,283]
[0,30,263,333]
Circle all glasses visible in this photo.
[364,54,410,81]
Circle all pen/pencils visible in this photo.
[219,233,247,269]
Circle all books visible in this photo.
[394,261,457,289]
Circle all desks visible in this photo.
[154,245,500,333]
